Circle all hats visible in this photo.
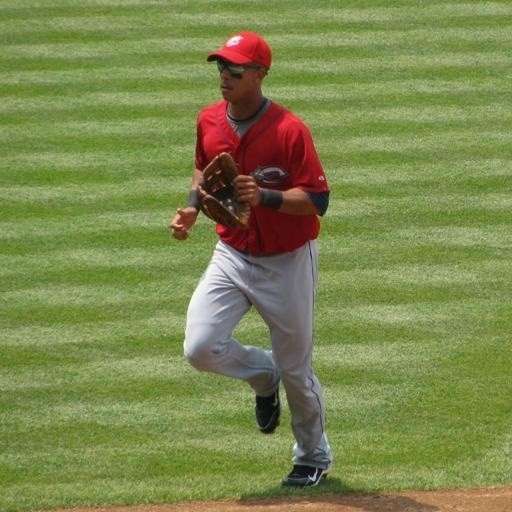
[207,31,272,68]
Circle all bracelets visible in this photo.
[259,185,282,210]
[186,189,203,212]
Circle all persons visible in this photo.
[169,31,335,488]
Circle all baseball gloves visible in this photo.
[197,152,250,230]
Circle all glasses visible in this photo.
[217,58,262,76]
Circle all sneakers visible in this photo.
[255,387,281,433]
[282,464,332,486]
